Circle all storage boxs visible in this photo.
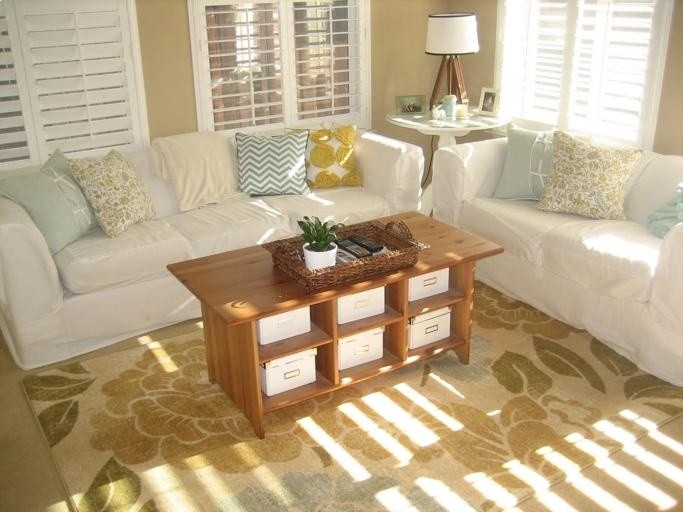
[253,305,311,347]
[334,325,386,372]
[406,305,452,352]
[407,264,450,304]
[336,285,385,327]
[255,347,319,398]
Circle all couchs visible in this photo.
[429,126,683,392]
[0,109,427,381]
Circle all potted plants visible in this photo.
[286,211,348,273]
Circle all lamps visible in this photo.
[422,12,483,114]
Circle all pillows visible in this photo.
[149,130,252,212]
[234,128,313,198]
[534,130,648,222]
[491,118,554,208]
[0,144,100,259]
[278,123,364,193]
[69,148,159,242]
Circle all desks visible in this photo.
[380,104,515,152]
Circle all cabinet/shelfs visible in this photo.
[163,209,505,441]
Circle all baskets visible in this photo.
[260,217,420,295]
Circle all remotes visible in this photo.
[348,234,384,252]
[335,239,373,259]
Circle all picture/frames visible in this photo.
[394,93,426,115]
[476,86,501,118]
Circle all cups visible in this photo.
[442,95,468,120]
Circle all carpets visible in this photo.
[20,278,682,512]
